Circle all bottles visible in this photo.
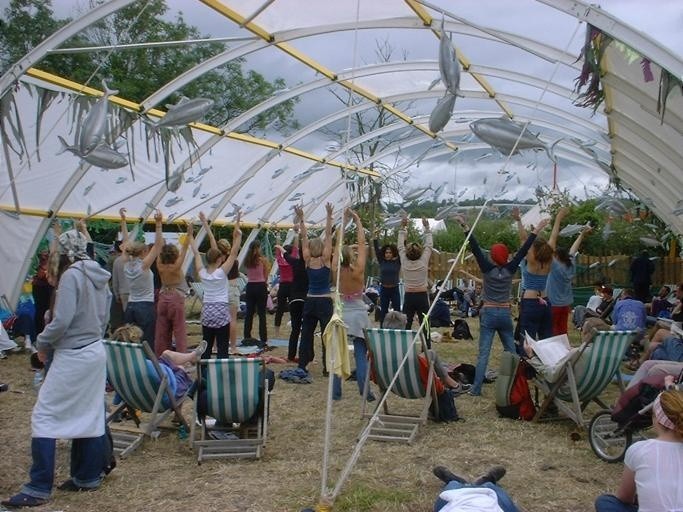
[33,370,41,385]
[25,335,32,349]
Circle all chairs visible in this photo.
[613,287,625,300]
[238,278,248,299]
[102,339,191,460]
[532,326,649,449]
[662,285,677,300]
[356,327,440,446]
[189,281,205,313]
[190,356,270,466]
[366,277,476,295]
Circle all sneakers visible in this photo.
[450,383,480,397]
[434,466,466,484]
[1,494,48,506]
[57,479,97,491]
[476,467,505,484]
[192,341,208,365]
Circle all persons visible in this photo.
[0,202,683,512]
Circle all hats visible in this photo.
[491,244,509,263]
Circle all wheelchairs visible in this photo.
[589,367,683,463]
